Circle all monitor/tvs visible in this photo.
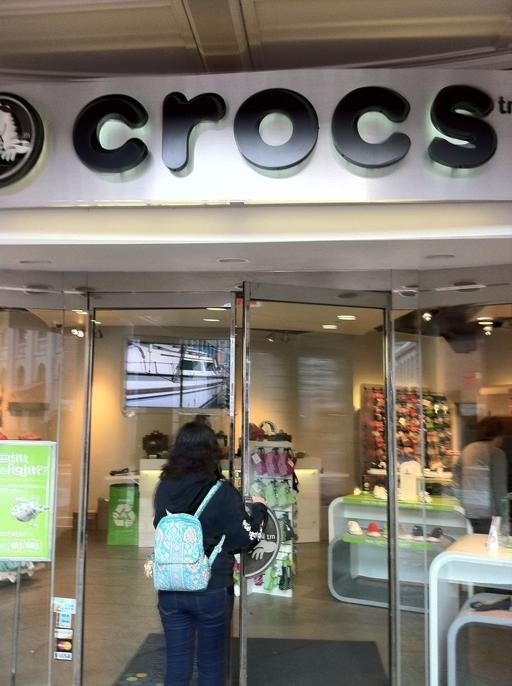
[122,333,229,415]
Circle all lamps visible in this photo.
[72,328,103,339]
[264,334,290,343]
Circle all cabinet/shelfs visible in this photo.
[233,439,296,599]
[328,493,473,615]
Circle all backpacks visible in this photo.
[153,479,227,592]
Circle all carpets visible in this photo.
[114,633,389,686]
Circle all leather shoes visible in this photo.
[469,596,511,611]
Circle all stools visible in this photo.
[447,593,512,686]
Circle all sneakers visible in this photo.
[348,520,364,536]
[367,522,381,538]
[412,526,424,542]
[427,527,444,543]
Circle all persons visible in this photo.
[234,420,260,456]
[450,412,511,595]
[151,418,269,684]
[195,412,216,428]
[247,540,277,561]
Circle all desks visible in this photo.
[429,533,512,686]
